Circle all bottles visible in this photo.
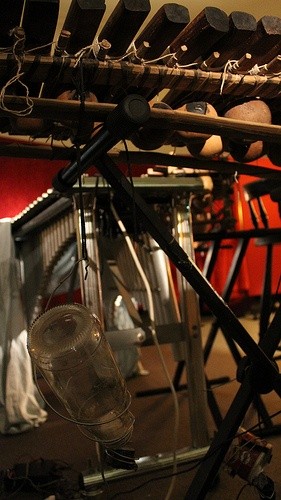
[27,303,135,448]
